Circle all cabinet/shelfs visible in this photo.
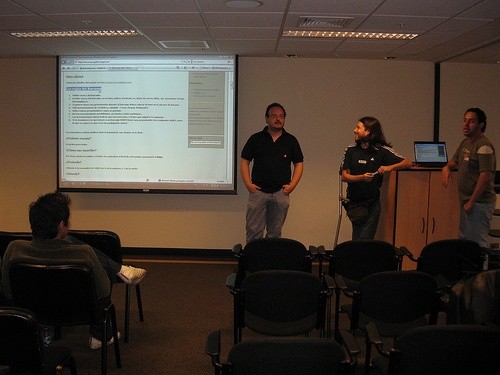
[373,166,459,271]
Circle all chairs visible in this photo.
[0,230,144,375]
[205,237,500,375]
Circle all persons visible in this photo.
[240,102,304,245]
[440,107,497,270]
[339,116,412,241]
[3,192,147,350]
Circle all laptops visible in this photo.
[414,141,449,168]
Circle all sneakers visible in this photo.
[117,264,147,287]
[89,331,121,349]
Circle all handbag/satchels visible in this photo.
[346,203,369,224]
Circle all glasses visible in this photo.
[271,114,284,118]
